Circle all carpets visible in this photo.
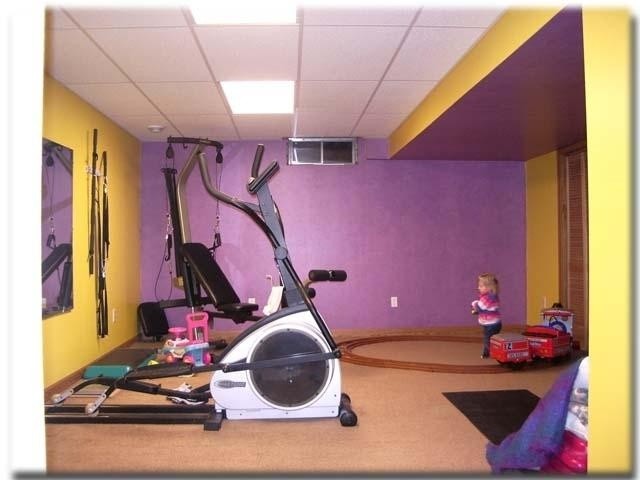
[443,390,540,444]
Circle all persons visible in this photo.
[470,273,502,359]
